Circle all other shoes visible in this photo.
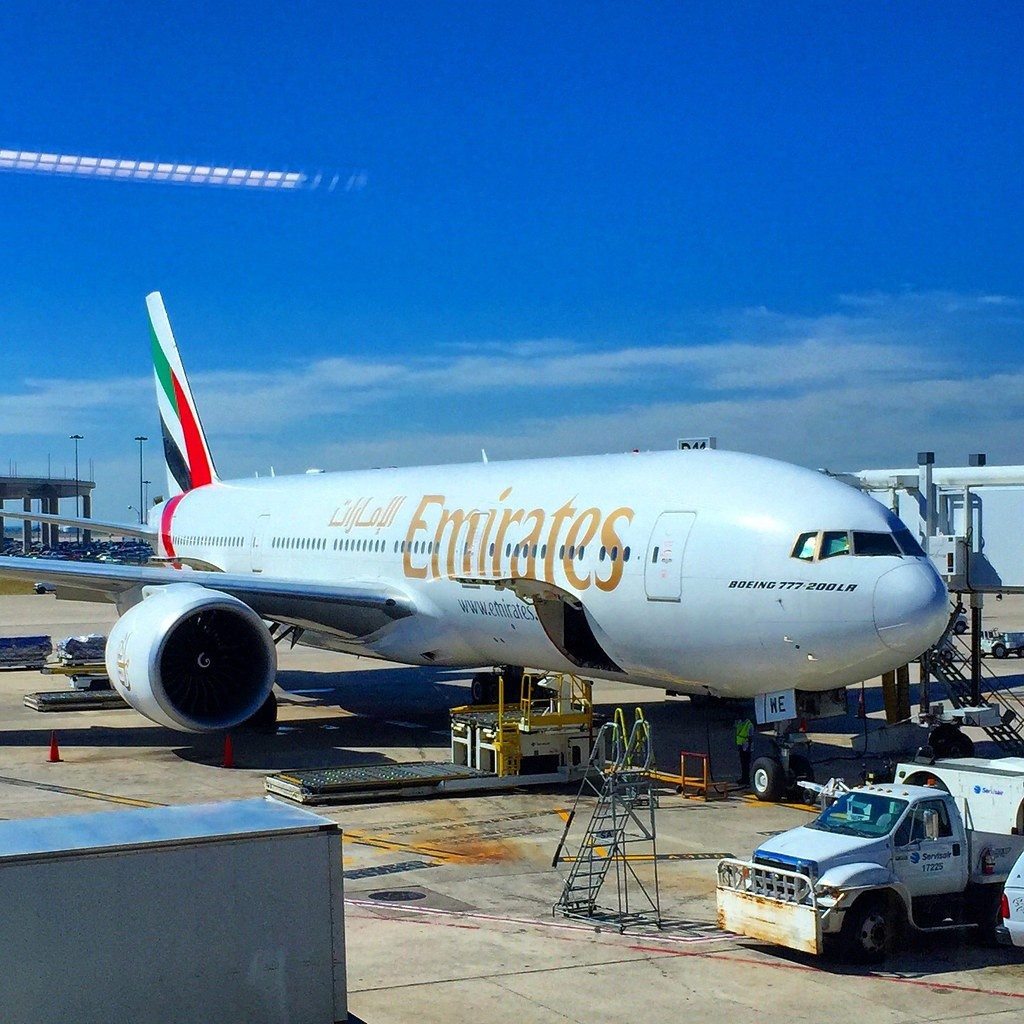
[735,779,750,788]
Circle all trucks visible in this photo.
[715,755,1024,967]
[977,628,1024,657]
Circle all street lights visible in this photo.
[70,434,84,536]
[142,481,152,522]
[127,505,142,563]
[134,436,147,523]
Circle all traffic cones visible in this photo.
[219,735,238,767]
[46,729,64,763]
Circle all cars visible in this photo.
[952,613,971,633]
[993,849,1024,950]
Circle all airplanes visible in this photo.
[1,293,1024,735]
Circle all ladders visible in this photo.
[550,719,662,934]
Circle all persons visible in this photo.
[783,718,815,784]
[732,711,754,785]
